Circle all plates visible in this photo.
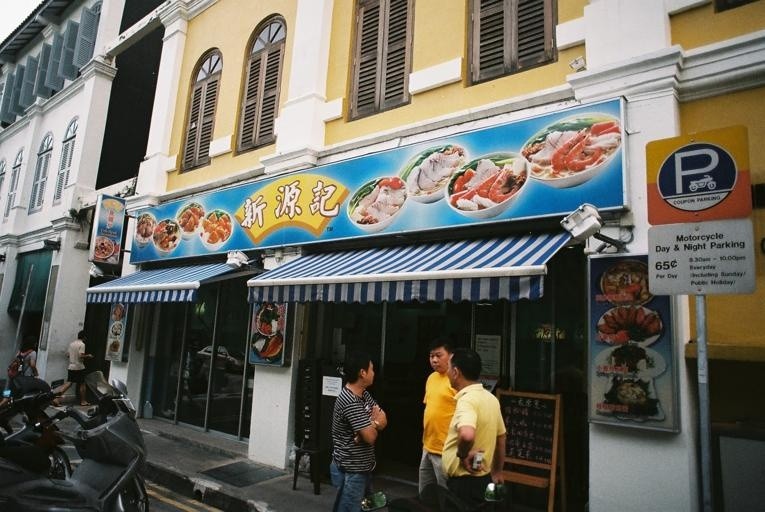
[110,322,123,338]
[152,218,183,257]
[109,338,121,357]
[255,304,282,337]
[597,307,663,349]
[110,302,126,323]
[252,330,283,363]
[601,258,654,308]
[602,375,666,420]
[592,344,668,381]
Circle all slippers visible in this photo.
[53,398,62,407]
[81,402,91,406]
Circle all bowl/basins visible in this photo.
[399,144,467,205]
[346,176,410,234]
[94,236,114,259]
[198,209,233,251]
[521,111,623,188]
[135,212,157,249]
[442,151,529,218]
[176,202,205,242]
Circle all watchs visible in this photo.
[374,420,379,430]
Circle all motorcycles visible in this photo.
[1,372,151,512]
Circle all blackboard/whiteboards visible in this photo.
[496,388,561,470]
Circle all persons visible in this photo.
[329,353,387,512]
[418,335,458,495]
[0,336,39,408]
[50,330,95,407]
[442,347,507,512]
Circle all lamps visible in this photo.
[560,203,633,253]
[88,263,121,279]
[224,243,262,273]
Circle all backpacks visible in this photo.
[7,348,33,379]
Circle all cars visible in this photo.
[197,345,245,371]
